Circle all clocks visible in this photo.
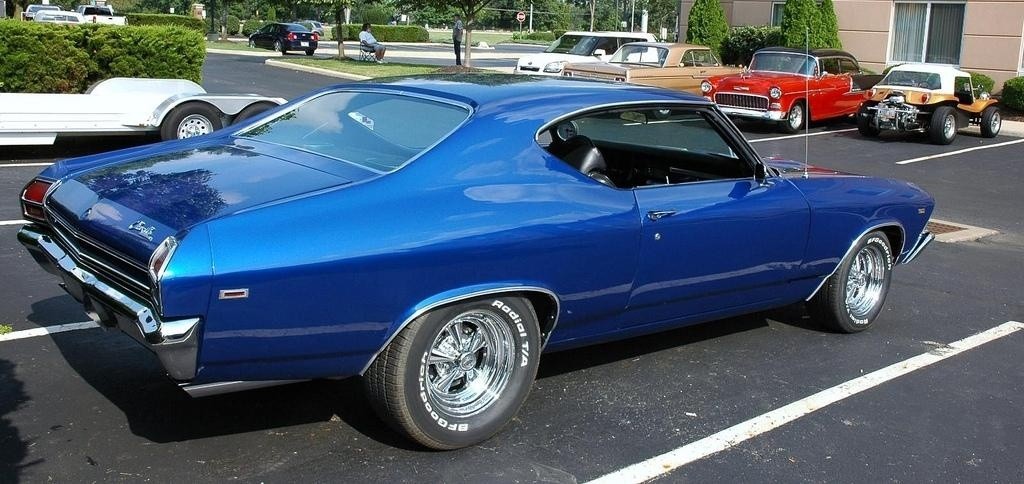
[556,119,579,141]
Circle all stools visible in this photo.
[359,42,376,63]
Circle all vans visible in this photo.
[293,19,324,37]
[33,9,86,26]
[513,30,661,78]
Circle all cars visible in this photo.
[559,41,745,97]
[0,75,289,149]
[16,69,935,450]
[855,62,1002,146]
[700,45,868,134]
[249,22,319,56]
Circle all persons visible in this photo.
[452,14,463,66]
[358,23,388,64]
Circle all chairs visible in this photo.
[552,134,616,187]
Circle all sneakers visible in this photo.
[376,59,389,64]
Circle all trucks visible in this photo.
[70,4,129,26]
